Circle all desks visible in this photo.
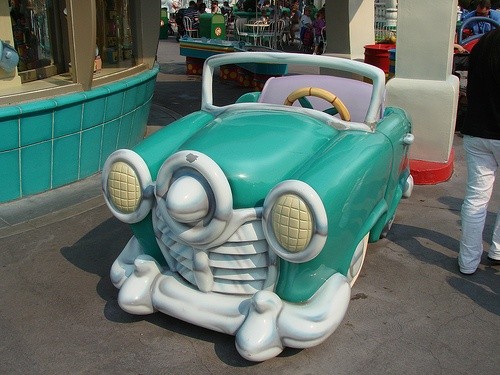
[244,24,270,45]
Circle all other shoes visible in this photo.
[486,256,500,263]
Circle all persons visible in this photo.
[454,0,500,54]
[168,0,327,55]
[458,29,500,273]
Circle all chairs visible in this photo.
[257,73,388,124]
[182,13,326,54]
